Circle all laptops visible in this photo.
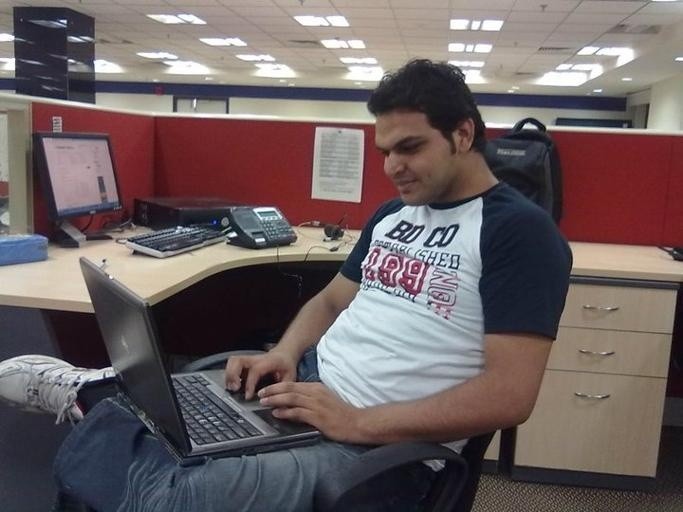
[80,256,323,469]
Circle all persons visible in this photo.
[0,60,573,512]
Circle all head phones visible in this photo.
[324,223,345,239]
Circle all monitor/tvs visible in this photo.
[32,130,123,249]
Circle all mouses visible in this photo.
[85,233,111,242]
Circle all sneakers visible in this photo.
[0,354,116,428]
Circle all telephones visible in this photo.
[227,205,298,249]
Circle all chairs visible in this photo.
[175,116,563,510]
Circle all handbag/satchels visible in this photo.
[482,118,562,226]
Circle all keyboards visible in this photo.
[123,224,226,259]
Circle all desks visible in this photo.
[0,221,683,511]
